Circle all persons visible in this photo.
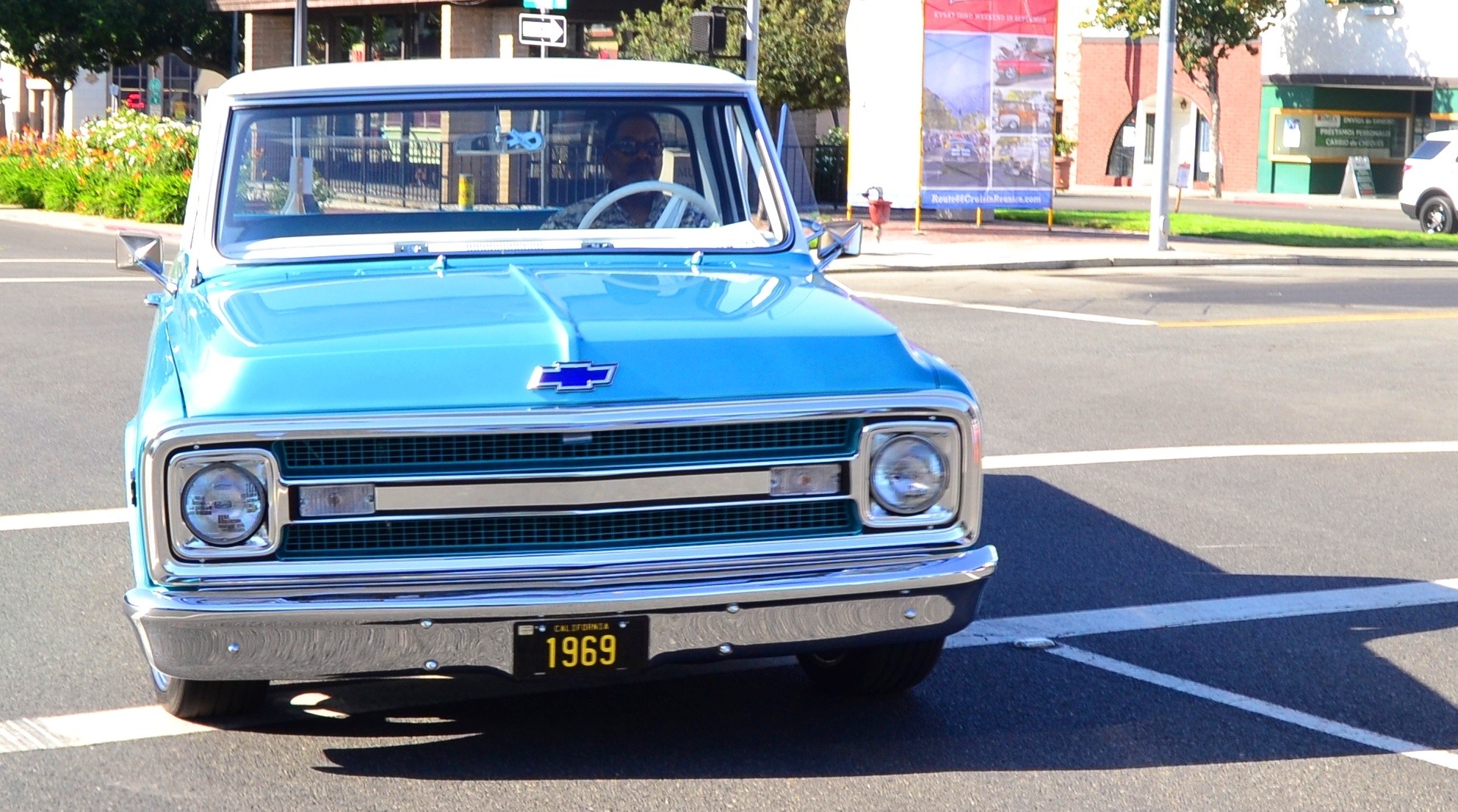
[979,133,987,160]
[538,113,712,228]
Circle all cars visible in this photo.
[116,56,997,722]
[1397,127,1458,238]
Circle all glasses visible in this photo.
[606,138,665,158]
